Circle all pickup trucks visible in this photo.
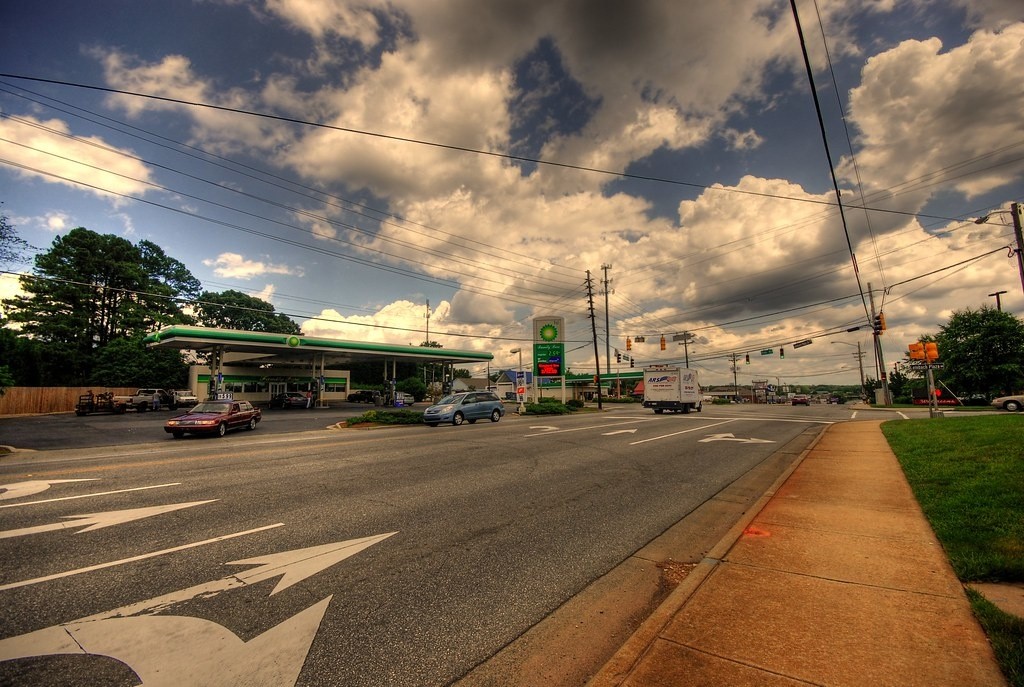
[115,388,168,412]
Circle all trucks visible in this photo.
[641,367,702,413]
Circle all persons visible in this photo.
[169,391,177,410]
[87,388,95,414]
[152,393,164,411]
[305,390,312,409]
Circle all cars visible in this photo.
[346,387,415,406]
[991,395,1024,412]
[791,395,810,407]
[163,400,263,437]
[423,390,505,426]
[268,392,307,410]
[171,389,198,405]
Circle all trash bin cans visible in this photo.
[375,397,383,407]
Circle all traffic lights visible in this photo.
[630,357,636,369]
[926,342,938,362]
[779,349,785,360]
[616,354,622,364]
[907,343,925,362]
[745,355,750,365]
[873,314,886,336]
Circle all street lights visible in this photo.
[830,341,865,394]
